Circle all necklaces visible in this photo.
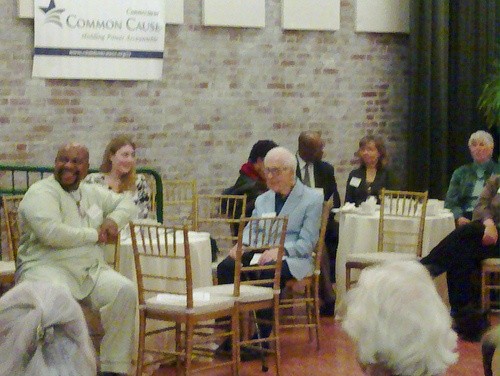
[365,169,376,194]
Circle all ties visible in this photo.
[303,164,311,187]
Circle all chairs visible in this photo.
[2,178,500,376]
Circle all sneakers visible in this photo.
[214,337,233,359]
[239,337,268,361]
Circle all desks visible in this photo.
[335,209,456,321]
[103,230,218,375]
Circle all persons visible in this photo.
[344,135,392,208]
[0,280,97,376]
[444,130,500,228]
[221,140,336,317]
[83,136,151,220]
[14,142,140,376]
[212,147,325,361]
[334,259,460,376]
[419,174,500,342]
[295,131,341,284]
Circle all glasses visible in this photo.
[262,167,289,175]
